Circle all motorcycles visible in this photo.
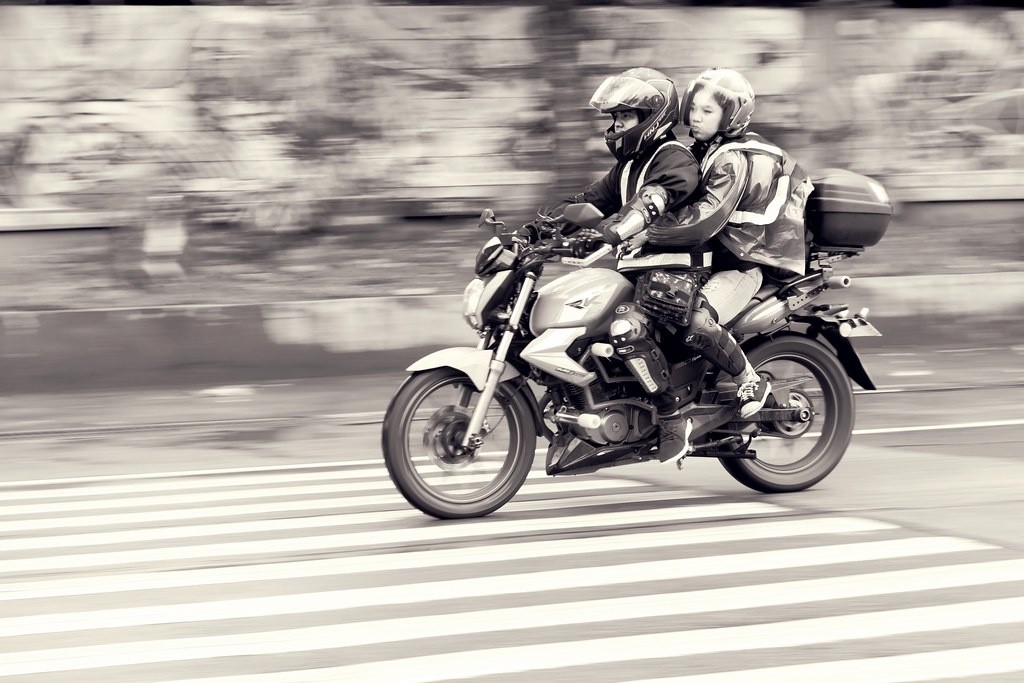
[380,169,894,517]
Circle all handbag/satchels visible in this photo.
[635,272,697,329]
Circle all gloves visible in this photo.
[569,233,607,259]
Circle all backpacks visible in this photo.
[700,131,782,216]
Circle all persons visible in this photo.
[517,67,705,466]
[620,66,816,418]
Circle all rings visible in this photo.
[627,239,632,246]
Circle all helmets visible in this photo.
[588,66,679,161]
[680,69,755,138]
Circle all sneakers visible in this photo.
[658,415,692,464]
[737,372,772,419]
